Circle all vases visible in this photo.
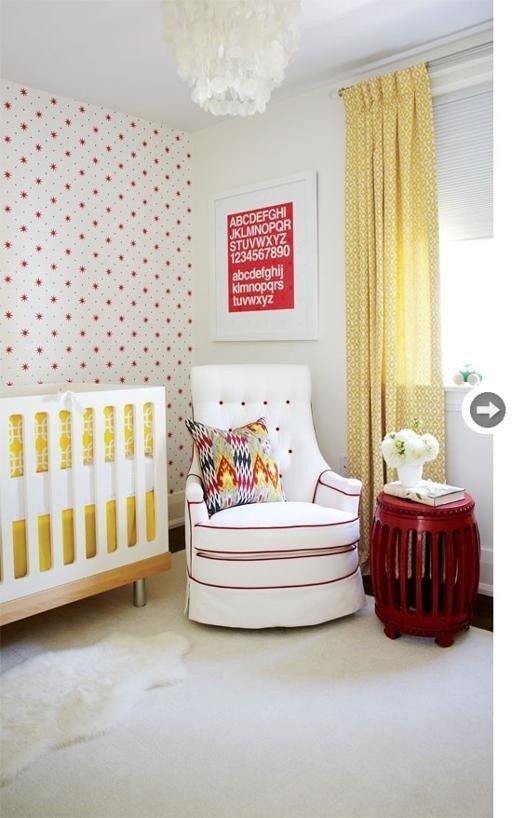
[396,464,423,488]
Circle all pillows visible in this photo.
[185,416,287,519]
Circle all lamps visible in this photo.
[159,0,302,117]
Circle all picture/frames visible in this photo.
[209,169,318,341]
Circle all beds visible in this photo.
[0,383,172,626]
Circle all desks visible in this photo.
[369,491,481,647]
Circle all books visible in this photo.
[383,479,466,506]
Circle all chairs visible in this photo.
[184,364,367,630]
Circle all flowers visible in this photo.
[382,416,440,467]
